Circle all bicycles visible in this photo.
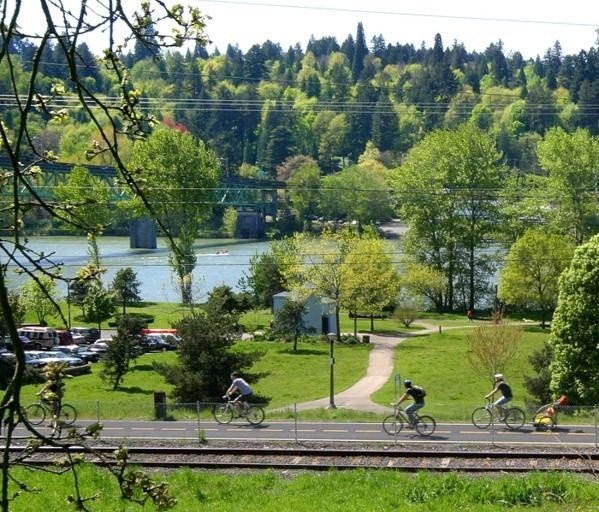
[472,397,525,429]
[383,403,436,437]
[212,396,265,425]
[25,391,77,427]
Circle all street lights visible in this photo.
[327,333,337,410]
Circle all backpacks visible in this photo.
[408,385,426,397]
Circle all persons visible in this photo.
[467,307,474,323]
[225,370,254,413]
[390,378,424,427]
[482,373,514,422]
[37,371,64,418]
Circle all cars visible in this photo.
[0,325,183,370]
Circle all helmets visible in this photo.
[403,380,411,385]
[494,373,504,379]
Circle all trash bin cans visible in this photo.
[363,336,369,343]
